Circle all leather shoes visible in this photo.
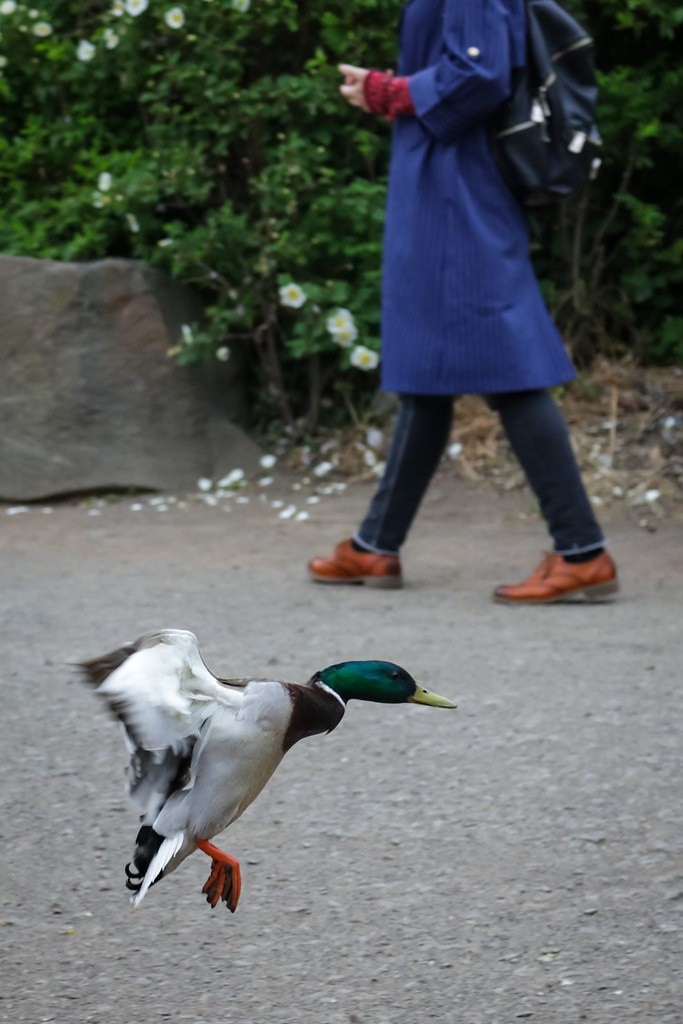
[492,552,619,603]
[310,540,403,589]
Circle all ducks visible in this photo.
[64,627,459,915]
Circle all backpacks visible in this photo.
[484,0,604,204]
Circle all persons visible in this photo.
[305,0,617,606]
